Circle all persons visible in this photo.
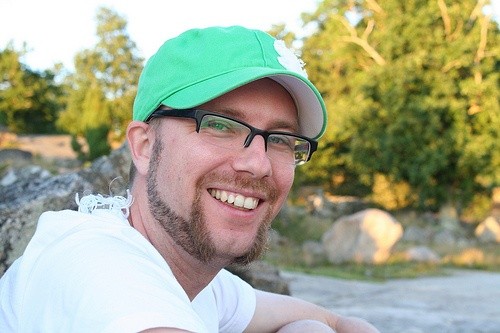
[0,26,382,331]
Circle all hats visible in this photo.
[127,24,328,143]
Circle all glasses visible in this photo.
[151,107,319,166]
